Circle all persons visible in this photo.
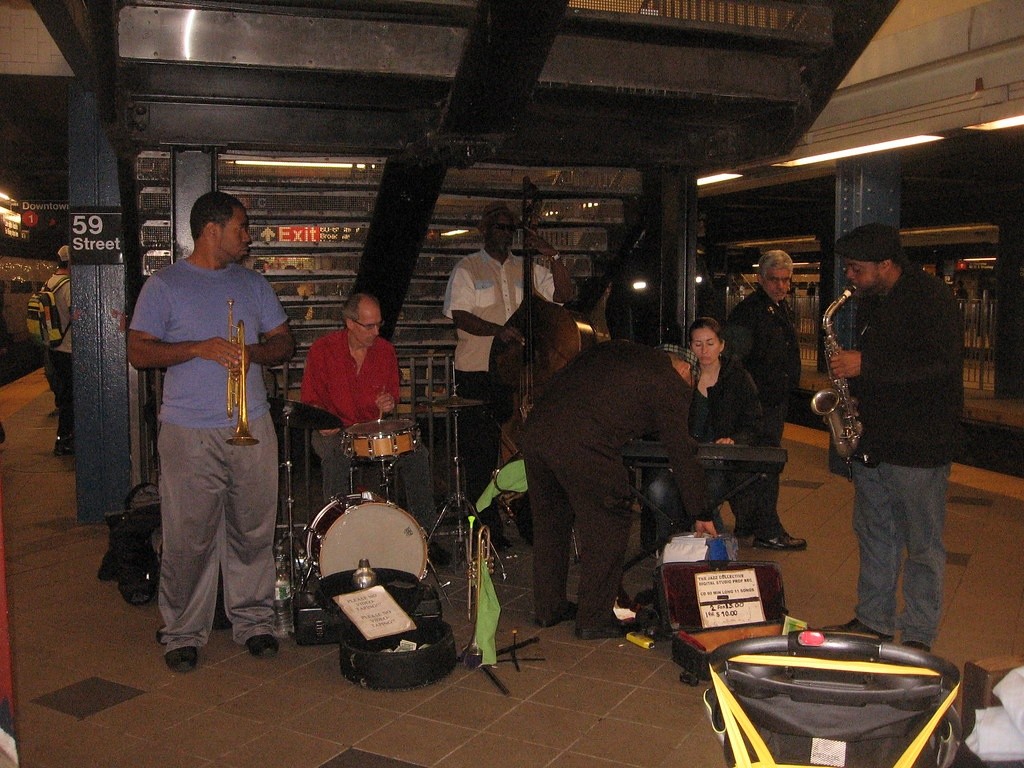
[299,292,438,534]
[127,192,298,673]
[41,245,74,455]
[723,247,810,549]
[515,339,710,641]
[649,318,764,540]
[823,221,964,653]
[439,202,576,552]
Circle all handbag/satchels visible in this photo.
[96,482,163,606]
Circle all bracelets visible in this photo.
[549,252,561,262]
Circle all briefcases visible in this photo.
[653,559,832,681]
[292,584,444,645]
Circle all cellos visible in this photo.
[490,179,597,564]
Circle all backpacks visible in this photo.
[25,276,71,348]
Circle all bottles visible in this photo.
[273,561,294,635]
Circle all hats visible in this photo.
[57,245,69,262]
[834,223,901,262]
[481,200,512,226]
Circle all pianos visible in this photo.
[620,439,789,577]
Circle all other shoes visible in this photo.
[164,646,198,672]
[535,601,578,627]
[490,535,512,552]
[817,618,894,643]
[521,537,533,547]
[901,641,930,652]
[576,618,640,640]
[246,634,279,658]
[54,434,74,458]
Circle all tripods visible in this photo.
[424,361,507,622]
[273,416,308,594]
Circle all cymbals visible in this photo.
[267,395,344,430]
[419,398,488,412]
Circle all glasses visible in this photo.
[487,222,515,232]
[347,317,385,331]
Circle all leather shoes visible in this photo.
[734,528,753,539]
[752,531,807,551]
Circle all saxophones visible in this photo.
[811,284,864,462]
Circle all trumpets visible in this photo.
[223,299,260,447]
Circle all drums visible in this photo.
[306,492,428,582]
[342,419,421,462]
